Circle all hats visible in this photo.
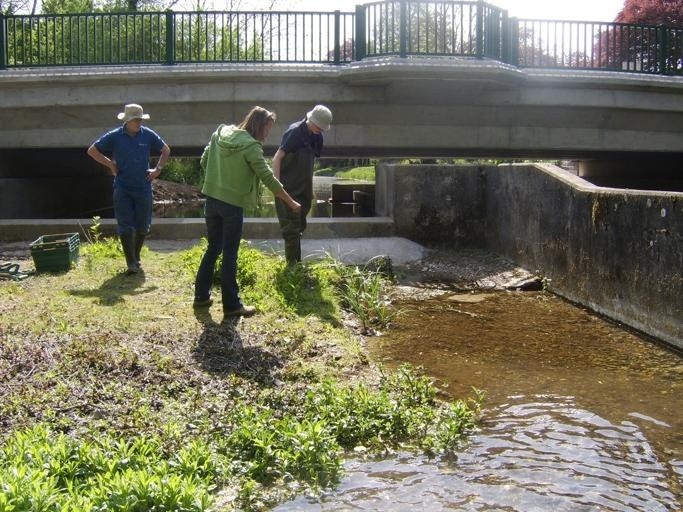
[116,104,150,122]
[306,105,332,130]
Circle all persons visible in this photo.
[87,104,171,273]
[193,106,302,316]
[271,104,333,263]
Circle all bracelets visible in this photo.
[156,167,161,171]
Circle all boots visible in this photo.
[119,228,146,275]
[284,236,302,267]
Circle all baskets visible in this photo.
[28,232,80,275]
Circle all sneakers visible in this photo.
[193,295,213,306]
[223,306,256,315]
